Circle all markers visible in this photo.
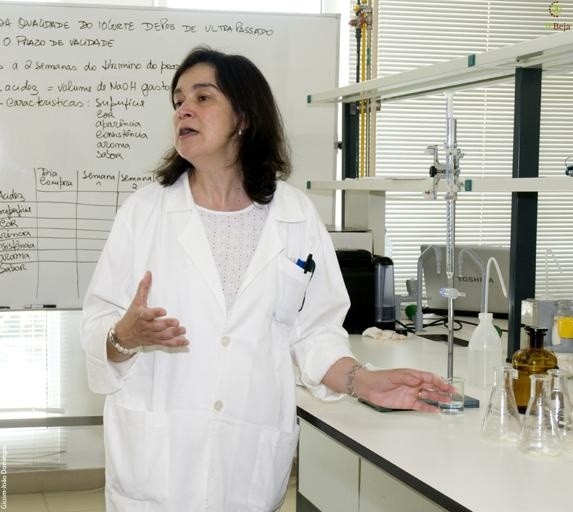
[0,305,56,310]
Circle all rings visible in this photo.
[416,390,422,400]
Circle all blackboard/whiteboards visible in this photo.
[0,1,340,312]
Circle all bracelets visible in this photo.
[345,364,361,398]
[108,324,137,356]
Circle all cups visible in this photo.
[439,377,465,416]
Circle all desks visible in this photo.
[293,332,572,511]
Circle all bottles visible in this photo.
[510,325,558,414]
[480,368,573,459]
[550,299,573,376]
[462,311,509,406]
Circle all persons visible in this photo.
[79,41,455,512]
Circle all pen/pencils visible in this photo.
[304,254,312,274]
